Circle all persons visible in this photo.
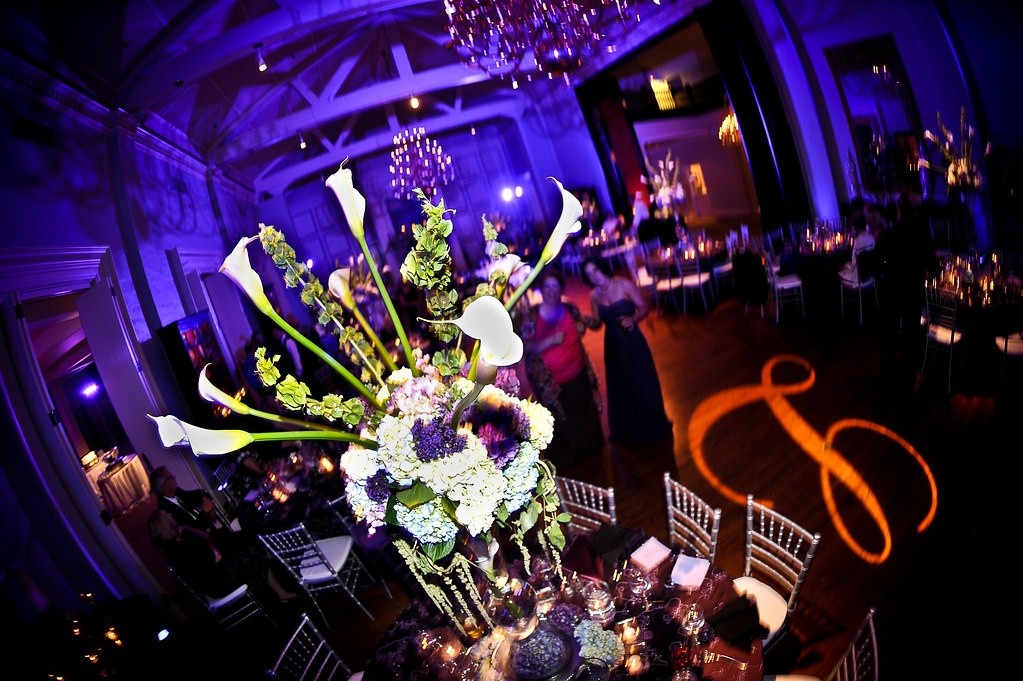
[147,509,296,603]
[579,257,673,442]
[241,326,321,396]
[150,465,216,530]
[507,237,566,294]
[863,132,922,192]
[520,270,606,466]
[399,283,422,331]
[838,216,876,282]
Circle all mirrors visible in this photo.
[824,33,930,197]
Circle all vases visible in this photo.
[393,465,564,641]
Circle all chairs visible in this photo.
[733,493,821,655]
[992,311,1023,386]
[555,225,882,326]
[267,612,366,681]
[325,486,412,600]
[664,472,722,565]
[762,607,878,681]
[260,524,375,627]
[920,284,963,395]
[167,567,269,641]
[553,477,617,537]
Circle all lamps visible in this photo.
[445,0,659,90]
[388,124,454,200]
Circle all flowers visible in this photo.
[144,162,585,542]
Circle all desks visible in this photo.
[930,268,1021,318]
[795,232,855,292]
[363,528,763,681]
[665,239,728,294]
[82,446,151,514]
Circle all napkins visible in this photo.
[630,537,711,591]
